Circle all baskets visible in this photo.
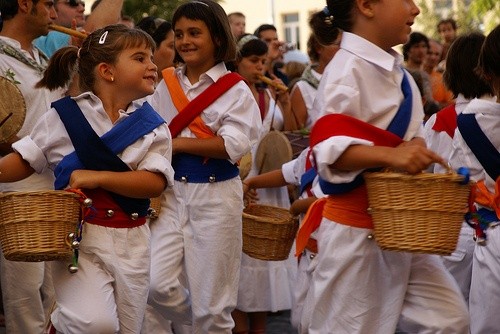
[242,203,301,261]
[145,197,160,218]
[363,149,474,256]
[0,189,80,263]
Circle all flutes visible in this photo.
[259,74,289,92]
[48,22,88,38]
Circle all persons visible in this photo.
[294,0,469,334]
[0,0,499,334]
[0,24,175,334]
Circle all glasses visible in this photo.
[56,0,85,8]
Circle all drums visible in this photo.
[255,128,309,175]
[1,76,27,143]
